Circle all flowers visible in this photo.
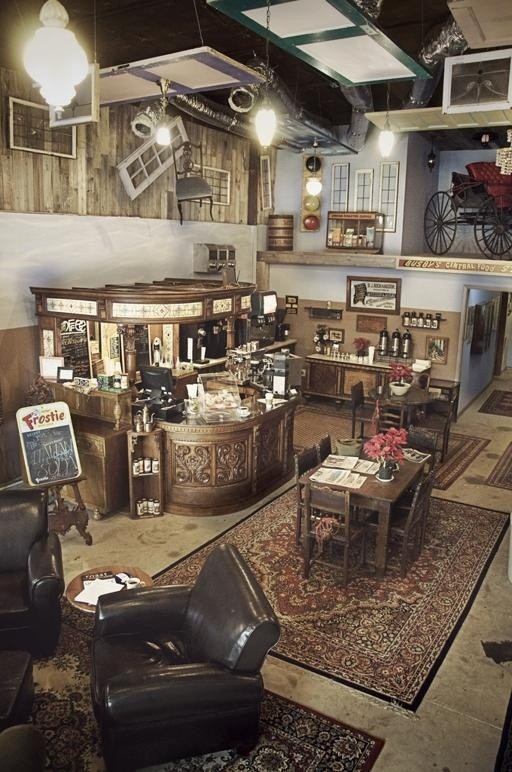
[312,324,329,353]
[388,362,413,386]
[353,337,370,353]
[359,427,410,467]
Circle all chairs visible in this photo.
[399,427,436,472]
[412,398,456,461]
[294,446,338,545]
[375,400,405,436]
[467,161,512,208]
[362,476,428,577]
[299,483,367,586]
[317,434,331,464]
[171,141,215,225]
[350,381,378,438]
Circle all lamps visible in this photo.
[305,137,323,197]
[379,83,394,159]
[22,0,89,107]
[426,133,436,172]
[150,80,175,147]
[250,1,278,149]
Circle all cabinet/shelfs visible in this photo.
[126,427,164,520]
[305,353,430,410]
[64,420,127,521]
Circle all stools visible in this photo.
[0,650,33,728]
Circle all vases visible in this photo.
[375,463,395,482]
[386,381,411,396]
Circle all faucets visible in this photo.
[415,373,430,389]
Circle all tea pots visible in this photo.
[136,404,155,429]
[183,396,201,419]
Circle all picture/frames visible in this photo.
[465,295,501,358]
[424,335,449,365]
[346,276,400,315]
[327,327,344,344]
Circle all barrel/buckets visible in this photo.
[335,438,362,457]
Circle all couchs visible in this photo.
[0,487,65,650]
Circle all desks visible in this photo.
[428,378,460,423]
[368,386,433,427]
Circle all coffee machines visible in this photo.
[247,291,278,349]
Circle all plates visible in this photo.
[375,473,394,483]
[241,412,251,418]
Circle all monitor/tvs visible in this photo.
[140,366,173,404]
[252,290,278,315]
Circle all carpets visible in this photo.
[479,389,512,417]
[0,594,387,772]
[420,424,491,490]
[152,483,509,713]
[485,441,512,491]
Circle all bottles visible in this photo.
[327,301,331,309]
[136,498,160,515]
[403,312,438,328]
[326,342,330,356]
[133,457,159,473]
[95,508,101,520]
[337,312,340,319]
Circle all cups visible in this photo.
[146,422,155,432]
[239,407,249,415]
[330,350,368,364]
[137,422,144,432]
[242,341,259,353]
[265,393,274,409]
[126,577,146,589]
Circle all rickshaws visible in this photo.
[423,161,512,261]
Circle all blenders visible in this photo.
[275,308,289,341]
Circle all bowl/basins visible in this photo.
[335,440,362,456]
[389,382,411,396]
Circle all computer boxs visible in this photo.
[132,397,185,418]
